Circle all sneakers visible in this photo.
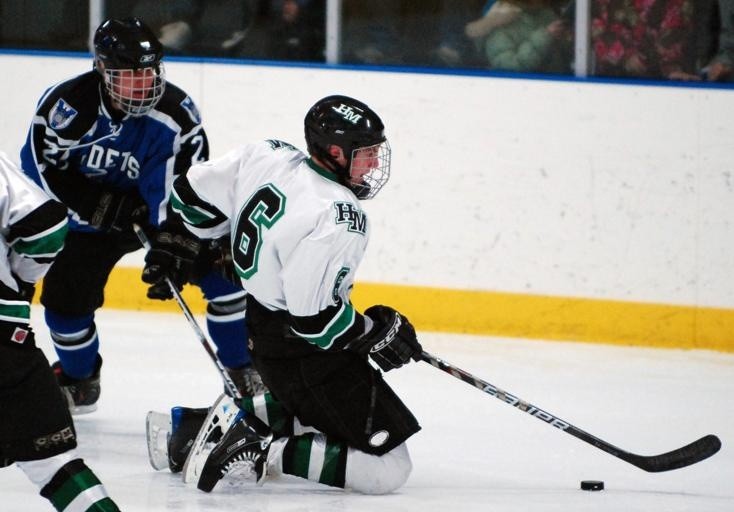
[52,354,275,492]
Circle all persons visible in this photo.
[256,0,326,62]
[164,93,427,497]
[467,0,734,83]
[0,145,130,512]
[15,15,271,411]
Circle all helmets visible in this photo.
[93,17,166,118]
[305,95,392,200]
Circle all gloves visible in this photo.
[80,186,147,240]
[141,222,200,300]
[362,306,421,371]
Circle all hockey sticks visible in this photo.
[416,353,721,473]
